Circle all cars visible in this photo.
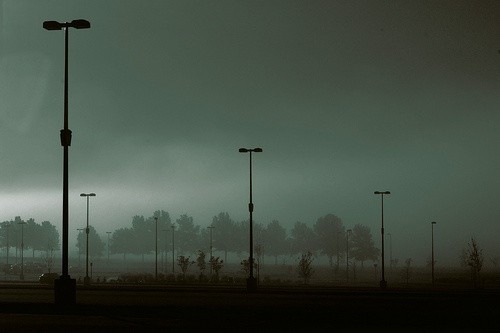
[37,272,59,284]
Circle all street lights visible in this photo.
[171,226,174,272]
[42,18,92,316]
[77,229,83,259]
[347,230,353,281]
[432,221,436,281]
[238,148,263,293]
[154,217,157,280]
[206,227,216,258]
[374,191,391,289]
[387,233,393,281]
[18,222,27,280]
[106,232,112,261]
[80,193,96,290]
